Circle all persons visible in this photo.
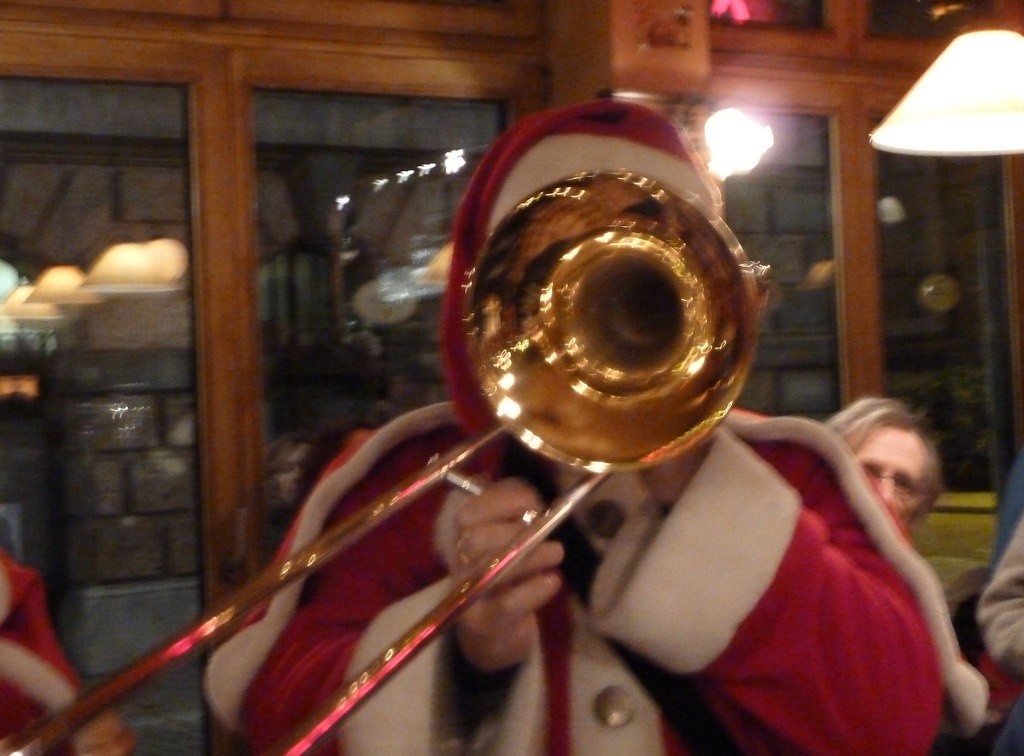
[203,99,987,756]
[826,391,940,539]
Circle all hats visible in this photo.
[441,100,722,480]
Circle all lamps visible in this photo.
[862,0,1024,157]
[0,285,65,320]
[23,263,101,306]
[77,237,191,295]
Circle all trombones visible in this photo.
[0,167,773,756]
